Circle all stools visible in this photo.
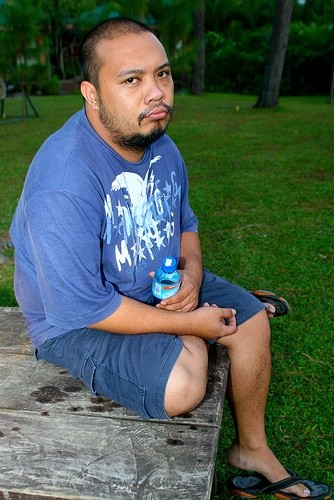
[0,305,233,500]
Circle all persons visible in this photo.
[7,17,333,500]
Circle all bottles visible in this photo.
[151,257,180,308]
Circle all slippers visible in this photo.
[245,289,292,320]
[227,466,333,500]
